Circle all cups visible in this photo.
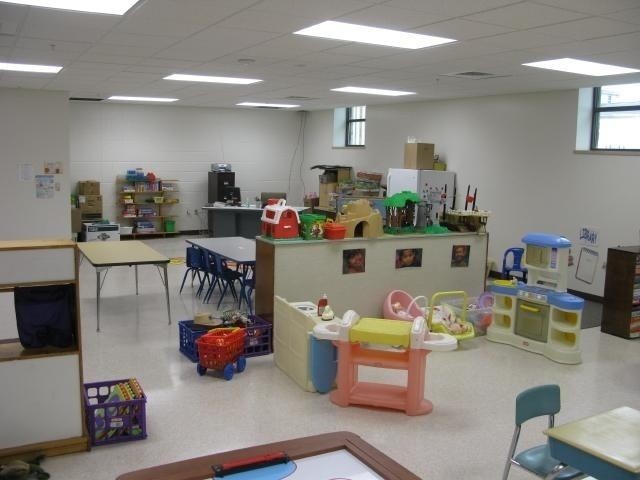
[434,162,446,172]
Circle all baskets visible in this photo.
[178,314,271,371]
[82,378,148,445]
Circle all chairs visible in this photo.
[180,246,243,310]
[502,384,590,480]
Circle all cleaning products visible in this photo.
[317,288,328,316]
[321,304,335,320]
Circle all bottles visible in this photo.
[254,192,260,208]
[317,290,327,316]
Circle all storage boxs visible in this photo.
[403,142,435,169]
[71,182,103,233]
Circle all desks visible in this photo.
[543,406,640,480]
[77,240,171,331]
[185,236,257,313]
[202,206,311,237]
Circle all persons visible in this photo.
[313,224,321,239]
[399,249,417,267]
[452,246,467,267]
[343,249,365,274]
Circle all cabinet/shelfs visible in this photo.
[118,179,180,235]
[0,240,89,467]
[601,245,640,339]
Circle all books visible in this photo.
[120,181,158,233]
[629,254,640,339]
[94,377,143,441]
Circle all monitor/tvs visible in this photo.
[222,187,241,206]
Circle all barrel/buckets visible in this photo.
[164,220,175,232]
[301,214,326,239]
